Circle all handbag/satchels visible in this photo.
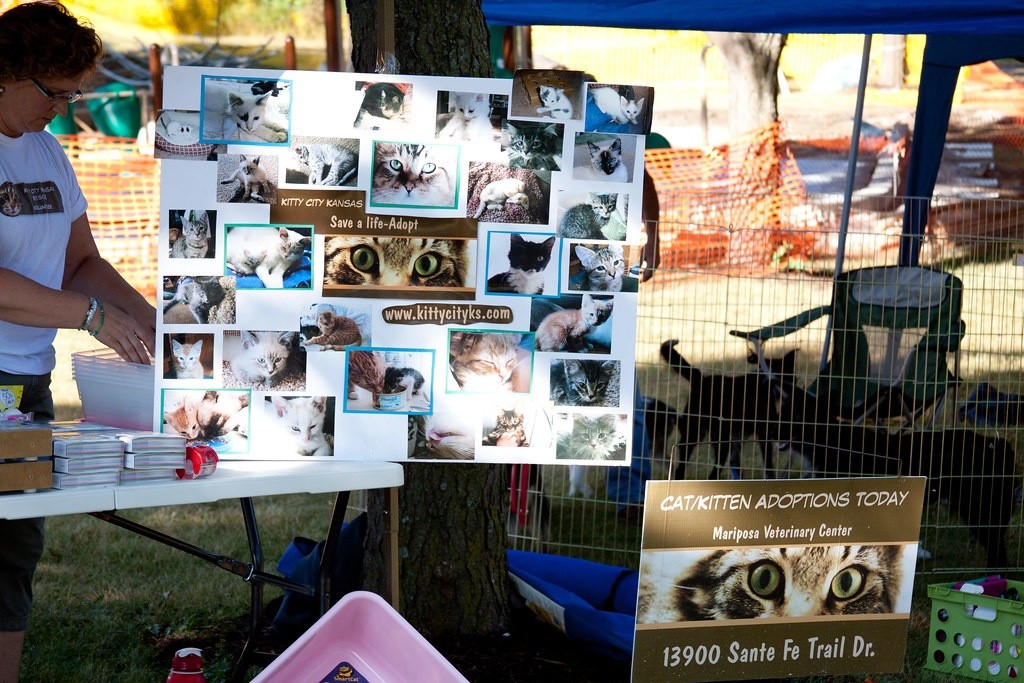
[263,512,369,636]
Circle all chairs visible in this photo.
[724,265,965,477]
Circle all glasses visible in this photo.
[28,76,82,104]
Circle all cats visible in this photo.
[156,66,657,465]
[635,546,909,620]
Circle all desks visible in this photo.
[0,420,405,683]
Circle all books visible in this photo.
[52,431,186,489]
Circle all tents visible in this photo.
[480,0,1024,368]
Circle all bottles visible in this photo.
[167,648,205,683]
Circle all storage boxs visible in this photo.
[71,344,161,432]
[923,576,1024,683]
[0,428,53,494]
[246,590,472,683]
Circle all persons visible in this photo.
[641,168,661,283]
[0,0,157,683]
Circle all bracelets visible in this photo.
[77,297,105,336]
